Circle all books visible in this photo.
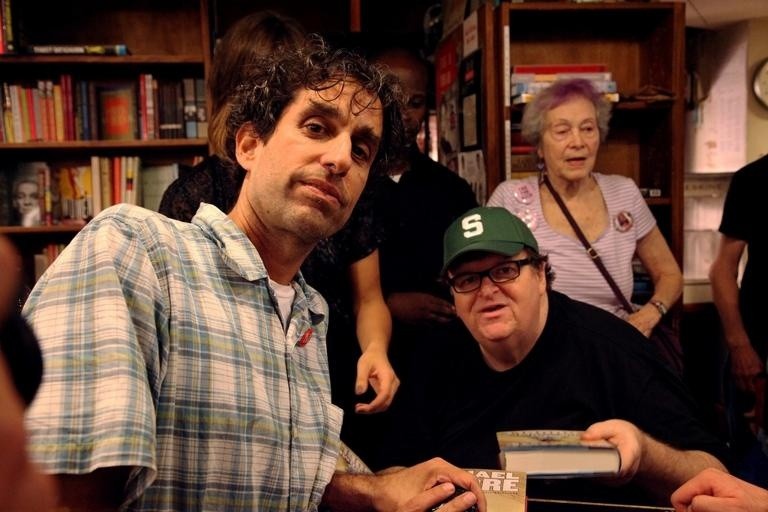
[1,74,208,144]
[512,65,621,180]
[1,156,140,227]
[496,429,622,482]
[142,156,204,212]
[35,244,65,283]
[33,45,127,55]
[0,0,14,56]
[639,188,662,198]
[461,469,528,512]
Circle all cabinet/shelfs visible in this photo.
[0,0,212,320]
[432,1,687,314]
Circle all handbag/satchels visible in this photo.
[649,314,705,372]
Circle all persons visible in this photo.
[326,208,731,512]
[157,11,412,479]
[0,35,485,512]
[485,78,684,371]
[669,469,768,510]
[711,155,764,489]
[329,33,483,464]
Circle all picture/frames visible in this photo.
[455,77,485,151]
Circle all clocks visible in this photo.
[752,57,768,110]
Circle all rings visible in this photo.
[430,313,440,324]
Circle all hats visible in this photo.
[441,207,539,276]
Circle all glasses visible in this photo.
[447,258,531,293]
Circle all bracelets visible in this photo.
[649,298,670,321]
